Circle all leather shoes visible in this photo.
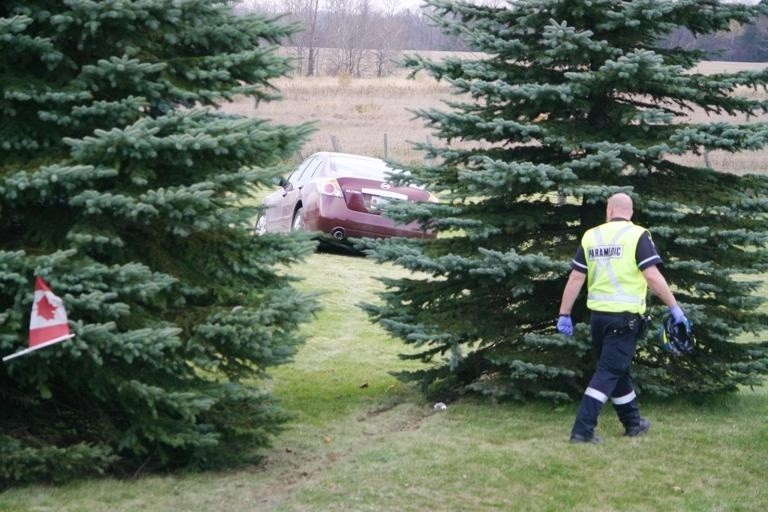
[626,418,650,436]
[570,436,603,445]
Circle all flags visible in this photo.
[29,276,69,346]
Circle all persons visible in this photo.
[557,193,692,445]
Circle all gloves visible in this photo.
[557,315,573,336]
[669,306,692,333]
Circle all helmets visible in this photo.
[658,314,696,358]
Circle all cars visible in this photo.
[246,150,441,243]
